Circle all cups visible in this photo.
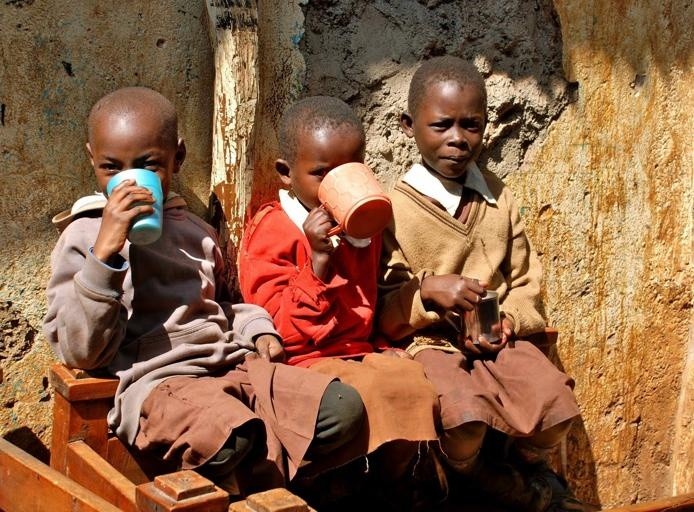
[460,290,502,344]
[318,162,393,239]
[107,169,164,246]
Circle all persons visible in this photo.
[43,85,365,500]
[375,56,582,512]
[238,95,449,503]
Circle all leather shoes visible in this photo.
[496,447,570,512]
